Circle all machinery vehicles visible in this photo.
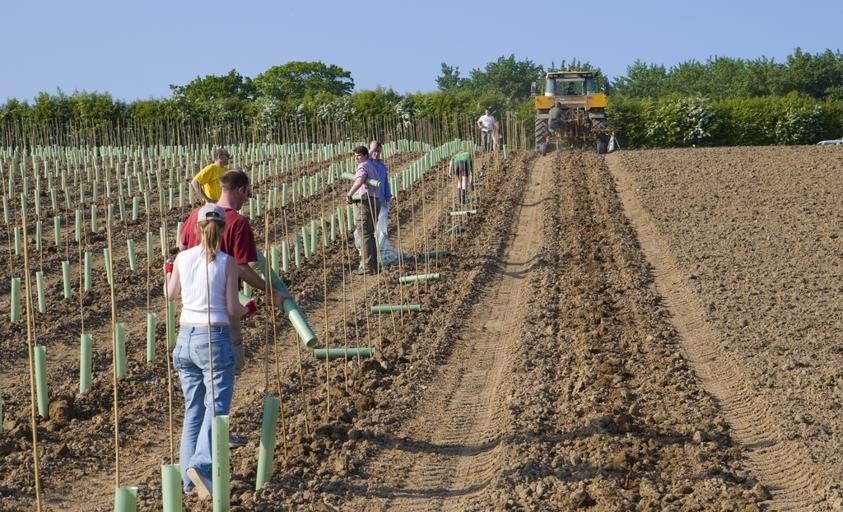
[530,68,611,154]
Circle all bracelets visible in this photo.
[346,195,352,200]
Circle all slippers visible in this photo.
[184,468,211,501]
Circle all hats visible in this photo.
[197,202,226,224]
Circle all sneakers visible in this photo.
[354,268,377,276]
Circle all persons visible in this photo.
[162,203,267,504]
[369,139,397,268]
[448,149,474,206]
[476,107,496,155]
[561,82,578,96]
[345,143,382,276]
[189,148,232,204]
[176,167,293,449]
[491,120,503,152]
